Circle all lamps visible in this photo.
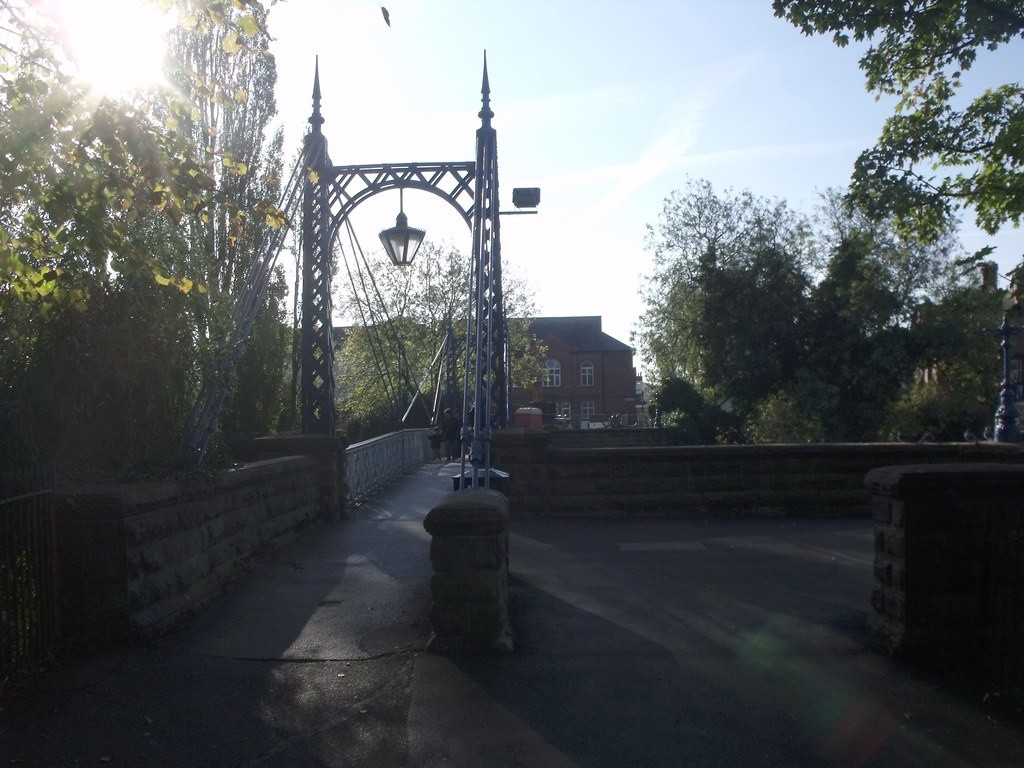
[380,182,424,267]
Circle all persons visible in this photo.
[469,405,485,433]
[438,407,456,464]
[427,426,444,462]
[453,409,463,458]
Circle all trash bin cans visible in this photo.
[452,467,511,581]
